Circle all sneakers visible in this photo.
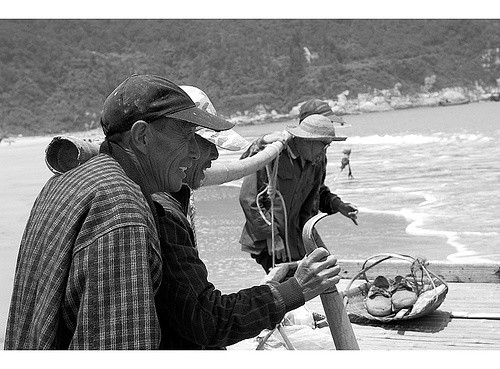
[366,276,393,316]
[391,275,417,308]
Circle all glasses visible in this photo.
[320,141,330,150]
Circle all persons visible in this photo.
[4,73,236,350]
[238,98,359,283]
[151,84,342,350]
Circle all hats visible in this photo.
[286,115,348,141]
[299,99,347,125]
[100,74,235,137]
[180,86,250,151]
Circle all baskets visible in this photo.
[340,253,448,320]
